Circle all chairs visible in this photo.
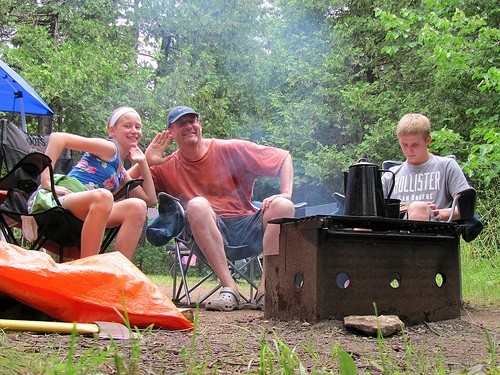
[0,153,144,263]
[332,155,484,243]
[158,193,308,307]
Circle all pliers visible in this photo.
[341,228,372,232]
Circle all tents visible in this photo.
[0,60,55,115]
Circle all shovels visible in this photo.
[0,319,130,339]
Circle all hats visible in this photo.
[167,106,199,128]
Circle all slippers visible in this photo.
[250,293,265,311]
[205,288,241,311]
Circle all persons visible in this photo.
[380,113,470,223]
[113,106,294,310]
[27,106,159,264]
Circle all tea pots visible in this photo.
[343,163,397,216]
